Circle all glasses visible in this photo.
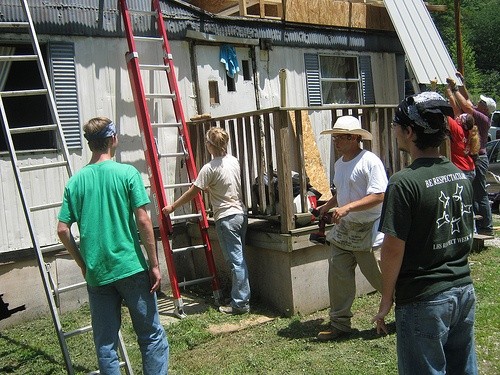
[332,135,352,142]
[390,120,415,129]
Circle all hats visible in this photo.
[320,116,373,140]
[480,95,496,113]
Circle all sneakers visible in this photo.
[219,306,250,315]
[318,326,343,339]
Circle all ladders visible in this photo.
[115,0,225,319]
[0,1,135,375]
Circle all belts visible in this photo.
[479,154,487,157]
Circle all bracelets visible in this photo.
[171,205,175,211]
[151,264,158,267]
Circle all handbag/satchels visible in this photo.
[326,217,382,252]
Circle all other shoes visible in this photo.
[478,230,493,235]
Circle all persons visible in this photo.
[162,126,250,315]
[371,92,478,375]
[430,77,476,181]
[315,116,389,340]
[446,72,496,236]
[57,117,170,375]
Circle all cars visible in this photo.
[483,111,500,139]
